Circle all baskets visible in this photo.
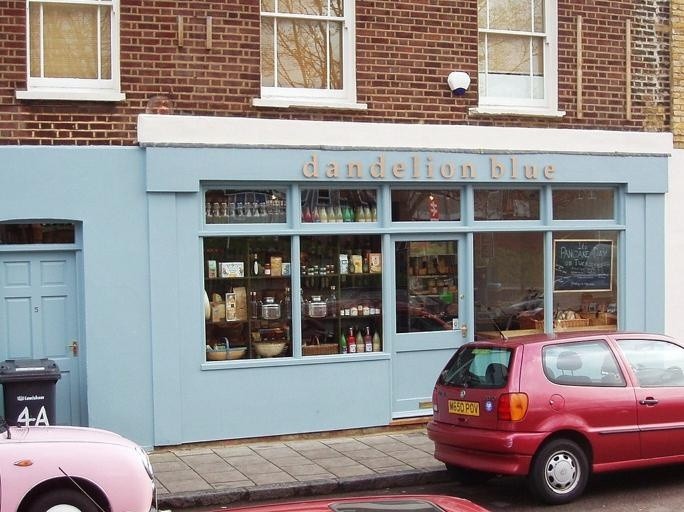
[302,334,339,356]
[206,334,247,360]
[576,309,618,326]
[534,316,590,330]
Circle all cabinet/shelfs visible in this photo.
[203,215,381,359]
[396,248,458,332]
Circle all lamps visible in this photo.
[448,71,471,95]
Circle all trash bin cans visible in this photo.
[0,359,61,426]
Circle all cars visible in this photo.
[427,333,684,505]
[1,416,157,512]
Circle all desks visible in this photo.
[477,325,617,342]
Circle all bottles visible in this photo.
[205,197,286,223]
[341,295,380,354]
[300,284,339,319]
[300,261,336,276]
[300,204,376,223]
[250,286,290,320]
[253,251,271,276]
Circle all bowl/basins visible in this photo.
[253,341,287,358]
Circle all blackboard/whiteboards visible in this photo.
[553,239,613,293]
